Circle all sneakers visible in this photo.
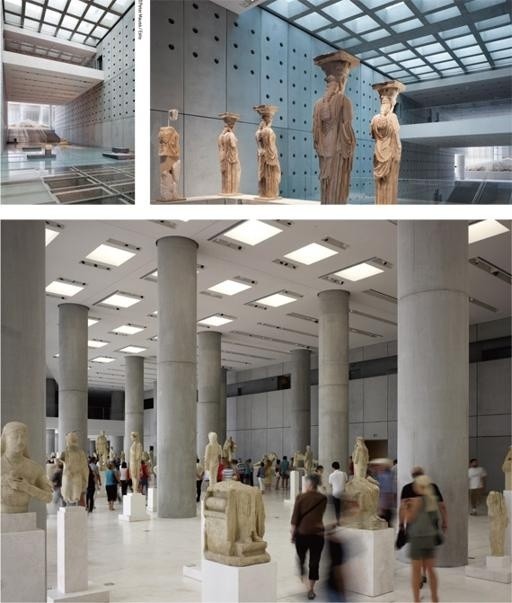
[471,511,477,515]
[420,576,426,588]
[308,590,315,599]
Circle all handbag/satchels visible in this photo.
[435,529,442,545]
[396,529,407,548]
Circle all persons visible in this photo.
[160,50,403,203]
[46,444,488,602]
[2,418,511,521]
